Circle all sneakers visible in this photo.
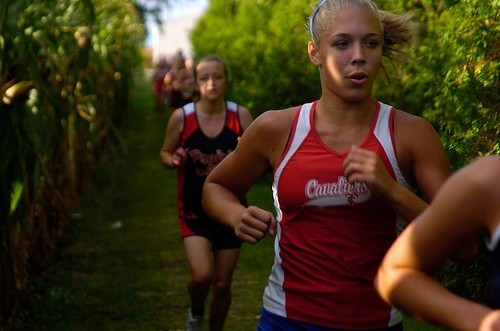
[186,308,202,331]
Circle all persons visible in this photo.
[198,1,455,331]
[159,53,256,330]
[373,151,500,331]
[154,47,199,115]
